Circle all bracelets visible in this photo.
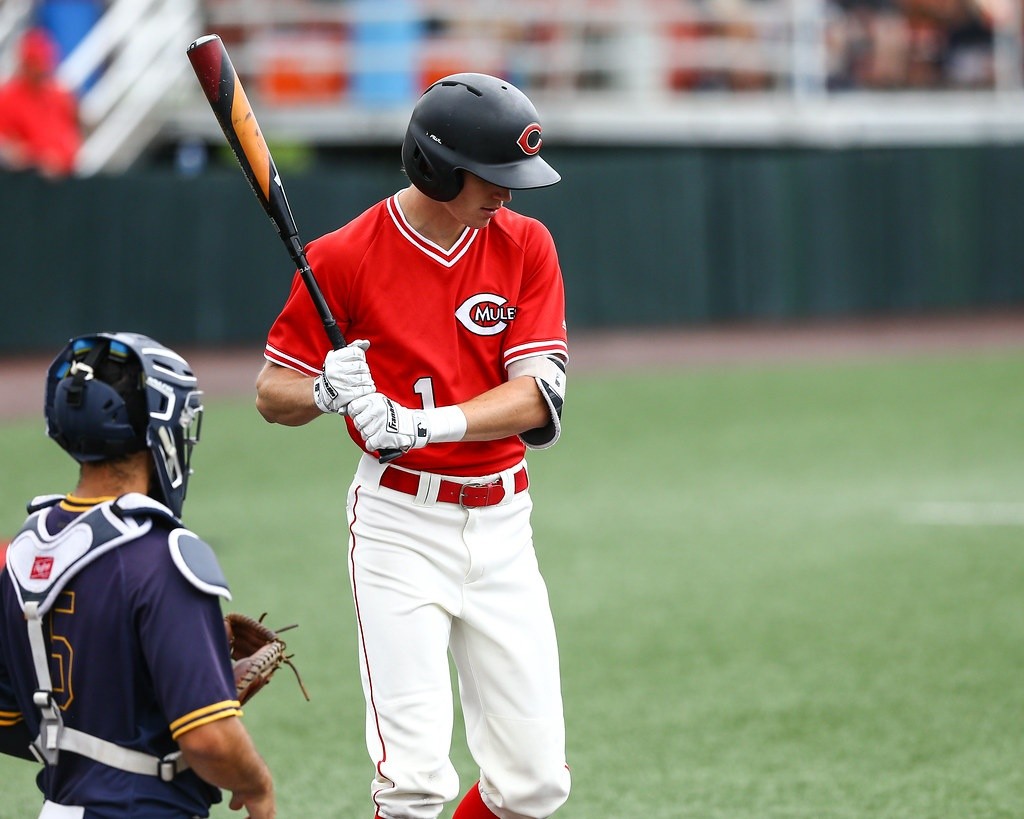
[423,405,468,444]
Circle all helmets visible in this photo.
[45,332,204,518]
[403,73,562,202]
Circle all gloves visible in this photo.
[314,339,376,413]
[347,393,431,452]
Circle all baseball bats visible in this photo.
[187,32,402,464]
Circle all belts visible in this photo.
[379,467,528,506]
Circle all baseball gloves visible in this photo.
[225,613,283,706]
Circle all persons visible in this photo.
[0,332,309,819]
[254,73,571,818]
